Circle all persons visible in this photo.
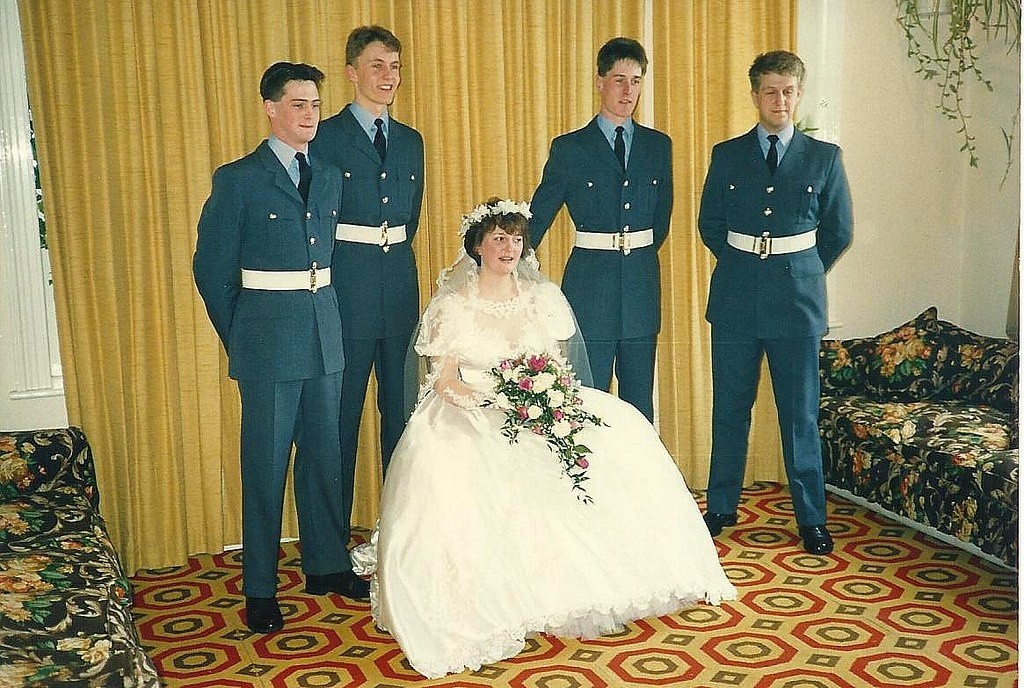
[527,38,672,425]
[698,51,853,555]
[350,198,739,681]
[310,27,424,545]
[193,62,370,631]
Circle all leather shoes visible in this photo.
[704,512,738,537]
[799,525,834,556]
[245,596,285,635]
[305,570,371,602]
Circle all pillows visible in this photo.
[843,307,966,403]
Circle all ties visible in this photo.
[295,152,311,205]
[767,135,780,175]
[614,126,626,170]
[372,118,387,164]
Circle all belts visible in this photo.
[726,230,817,260]
[575,228,654,256]
[240,268,331,294]
[335,224,408,248]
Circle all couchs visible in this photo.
[817,320,1019,574]
[0,426,162,688]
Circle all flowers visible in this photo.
[457,199,533,238]
[477,351,611,507]
